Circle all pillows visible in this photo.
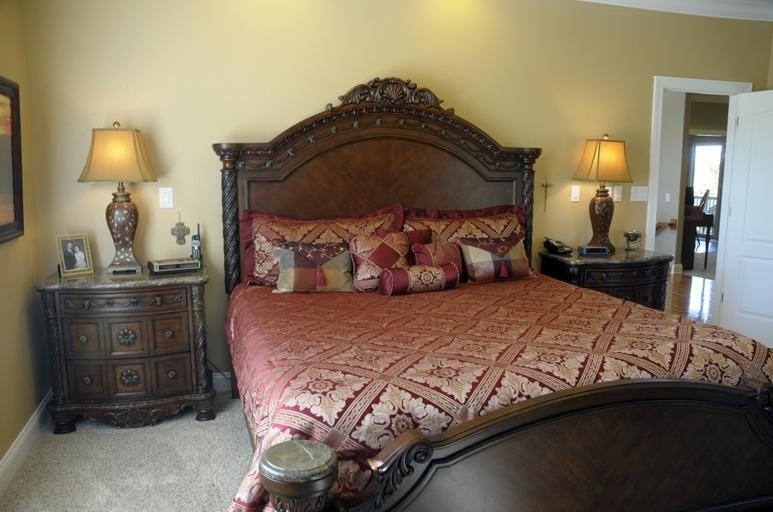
[237,203,536,295]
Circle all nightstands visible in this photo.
[538,245,674,313]
[36,265,215,434]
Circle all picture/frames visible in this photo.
[0,77,24,244]
[54,233,94,278]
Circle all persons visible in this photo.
[63,242,76,270]
[73,245,86,268]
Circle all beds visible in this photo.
[209,78,773,511]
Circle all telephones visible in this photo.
[543,236,573,254]
[192,224,203,260]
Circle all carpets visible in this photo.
[684,251,717,281]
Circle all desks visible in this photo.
[682,210,714,272]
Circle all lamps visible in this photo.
[572,133,633,254]
[75,123,159,275]
